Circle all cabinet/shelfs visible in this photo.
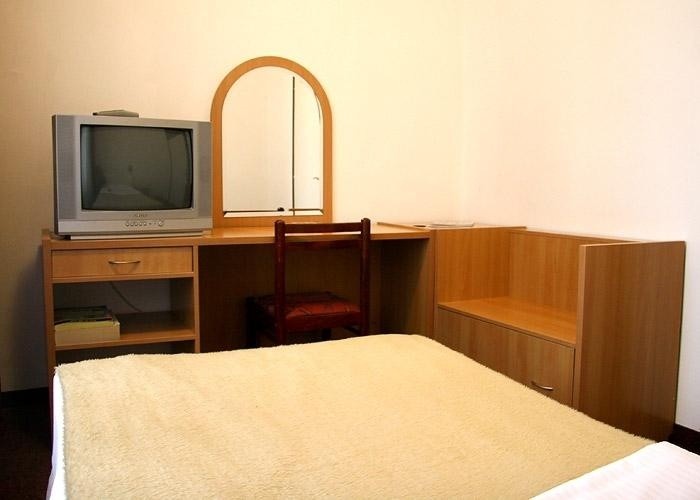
[432,227,686,441]
[45,233,198,438]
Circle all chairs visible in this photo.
[246,219,370,349]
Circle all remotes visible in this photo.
[92,109,139,118]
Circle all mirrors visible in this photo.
[211,56,332,227]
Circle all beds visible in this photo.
[46,333,699,500]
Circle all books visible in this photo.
[54,305,121,346]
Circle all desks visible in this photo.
[195,222,431,354]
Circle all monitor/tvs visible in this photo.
[52,113,212,240]
